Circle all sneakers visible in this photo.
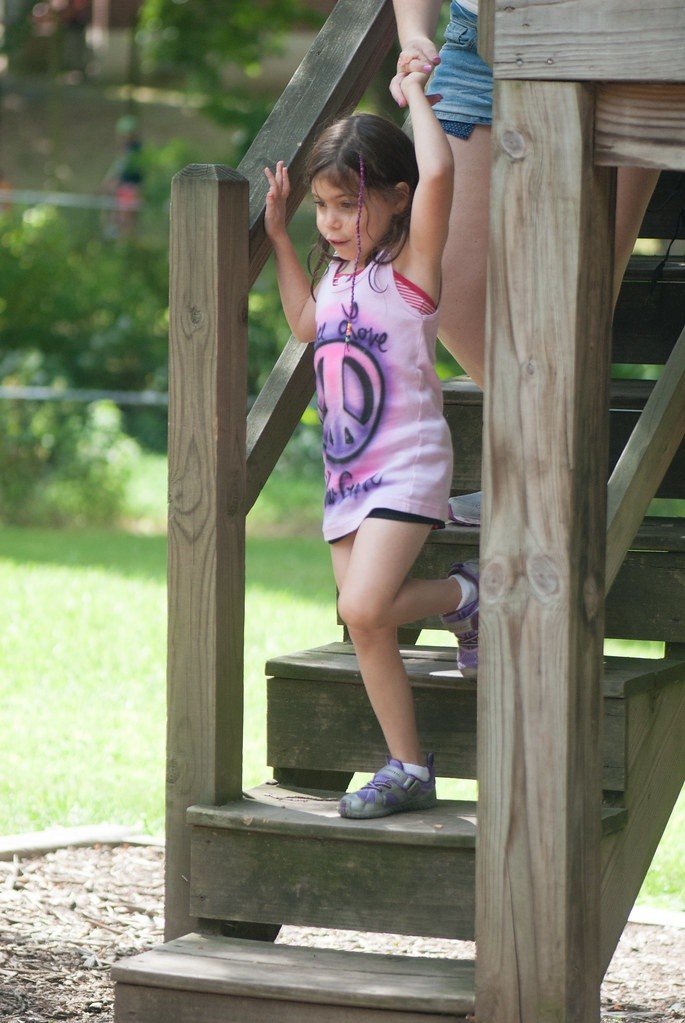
[439,557,478,679]
[337,752,438,817]
[448,491,483,526]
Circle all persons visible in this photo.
[264,48,481,819]
[390,0,663,526]
[103,113,147,236]
